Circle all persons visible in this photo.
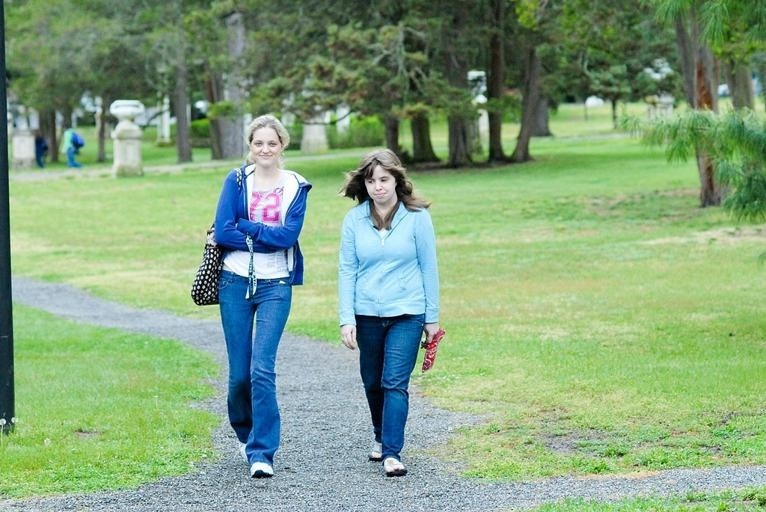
[337,146,441,477]
[35,130,48,168]
[61,123,82,167]
[212,112,313,478]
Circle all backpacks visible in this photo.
[70,132,84,148]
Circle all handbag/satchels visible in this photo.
[191,222,225,308]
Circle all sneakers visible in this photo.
[382,456,409,477]
[249,462,274,478]
[238,441,250,462]
[367,439,384,462]
